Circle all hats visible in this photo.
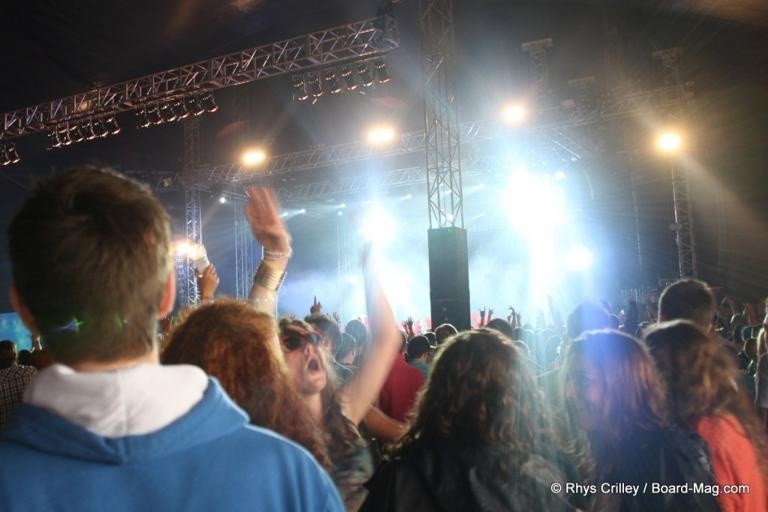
[407,335,437,350]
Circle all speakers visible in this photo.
[428,226,470,299]
[431,299,471,332]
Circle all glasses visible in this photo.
[280,330,324,352]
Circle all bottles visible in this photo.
[189,243,211,274]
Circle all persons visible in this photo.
[644,320,768,512]
[157,184,459,512]
[0,169,343,511]
[1,337,53,431]
[713,289,767,422]
[474,292,659,389]
[658,279,716,332]
[355,326,584,511]
[563,329,719,511]
